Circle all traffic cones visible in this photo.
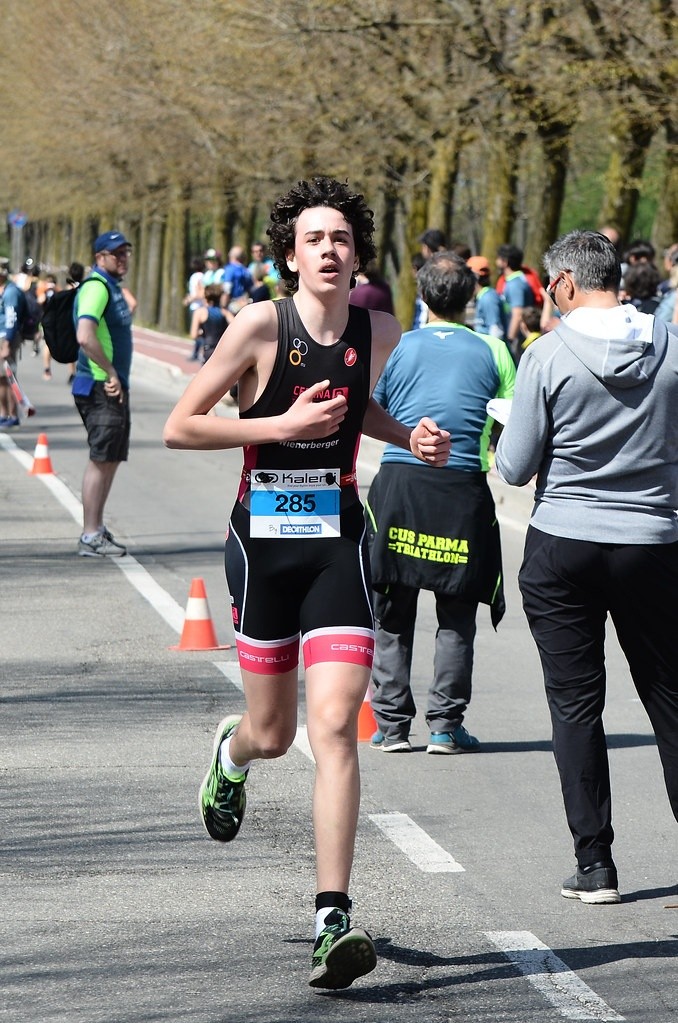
[27,433,58,475]
[168,577,231,651]
[356,685,379,742]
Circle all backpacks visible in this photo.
[1,283,40,340]
[40,278,112,364]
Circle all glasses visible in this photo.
[102,249,133,257]
[546,269,572,307]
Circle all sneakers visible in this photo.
[426,724,480,755]
[369,728,412,753]
[309,908,376,990]
[76,524,127,558]
[198,714,250,846]
[561,859,621,905]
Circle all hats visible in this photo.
[202,248,222,260]
[466,256,490,276]
[0,256,13,276]
[94,231,132,252]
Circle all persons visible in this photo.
[597,226,678,325]
[7,261,136,385]
[349,262,395,315]
[363,250,516,754]
[41,232,136,558]
[494,229,678,904]
[413,229,563,455]
[0,257,24,427]
[163,178,451,989]
[182,242,292,404]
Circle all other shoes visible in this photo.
[43,368,52,381]
[66,373,75,385]
[0,416,21,429]
